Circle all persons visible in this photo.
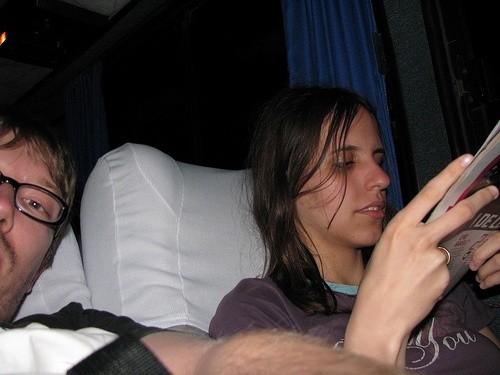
[208,81,500,375]
[0,98,418,375]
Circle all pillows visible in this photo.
[11,218,95,325]
[81,143,273,336]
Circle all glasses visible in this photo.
[0,171,69,225]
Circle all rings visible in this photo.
[438,246,451,264]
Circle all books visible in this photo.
[425,120,500,301]
